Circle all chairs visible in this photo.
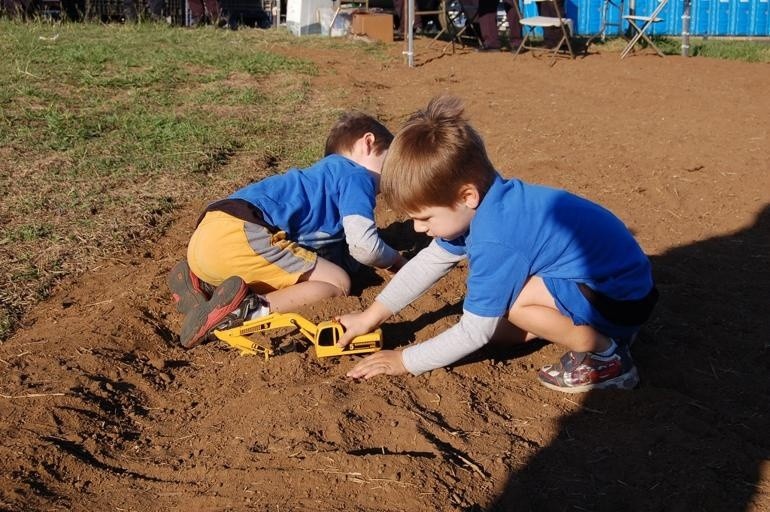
[620,1,669,60]
[512,0,574,66]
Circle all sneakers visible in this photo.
[536,340,641,394]
[178,274,261,350]
[163,260,209,315]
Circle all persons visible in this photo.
[165,112,411,351]
[388,0,425,41]
[327,91,662,395]
[415,0,485,47]
[534,0,573,55]
[470,0,527,54]
[186,0,224,28]
[118,1,166,25]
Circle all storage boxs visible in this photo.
[318,9,394,45]
